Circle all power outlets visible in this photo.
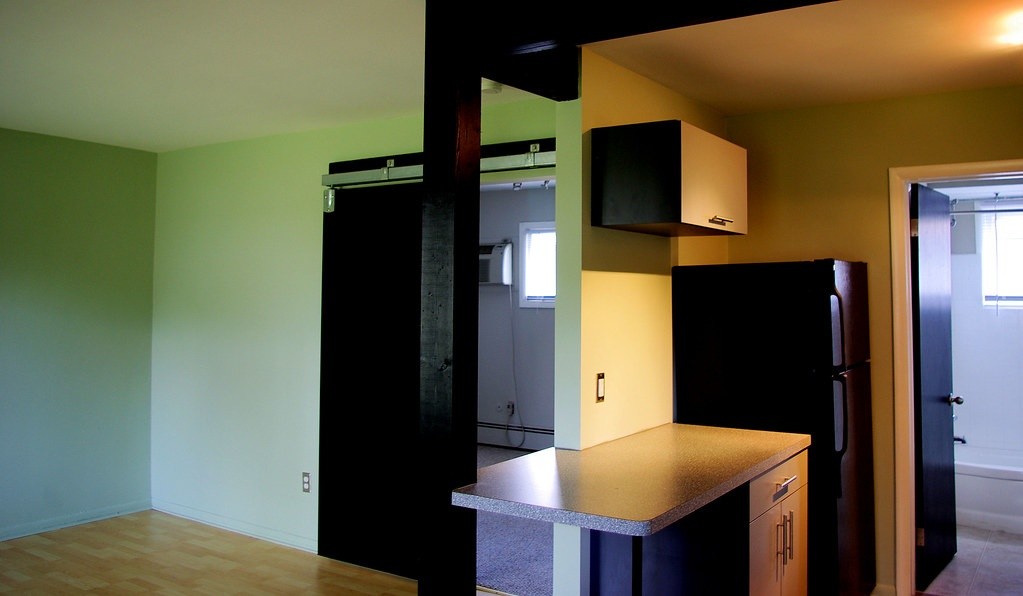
[597,374,606,402]
[506,401,513,415]
[302,472,311,493]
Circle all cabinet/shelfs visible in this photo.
[590,119,750,236]
[597,448,810,596]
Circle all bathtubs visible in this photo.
[954,445,1023,534]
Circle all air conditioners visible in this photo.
[478,243,513,287]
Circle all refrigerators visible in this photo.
[671,258,870,596]
